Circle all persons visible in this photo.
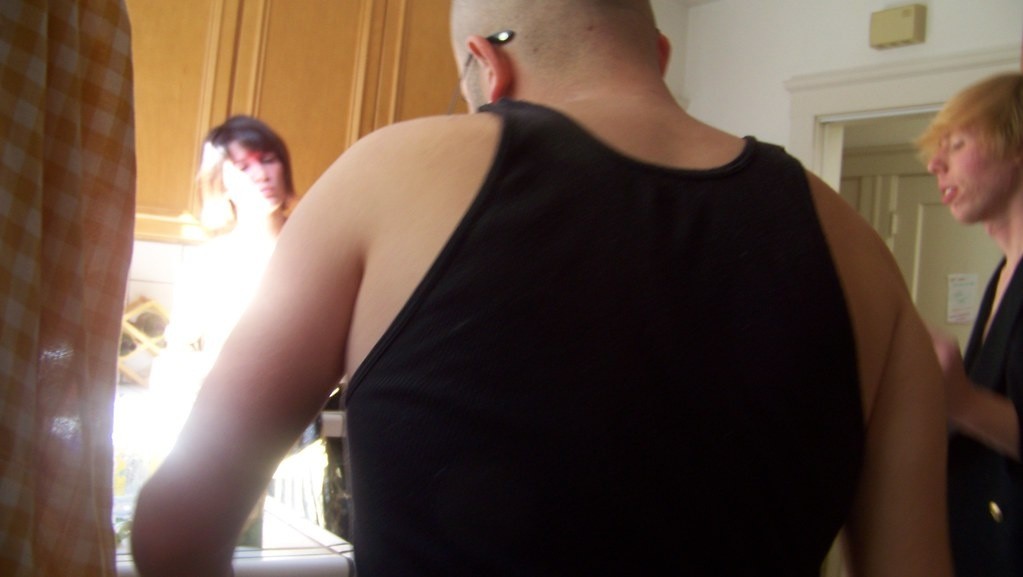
[922,71,1023,577]
[191,115,302,237]
[131,0,953,577]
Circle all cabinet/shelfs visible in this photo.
[126,0,467,242]
